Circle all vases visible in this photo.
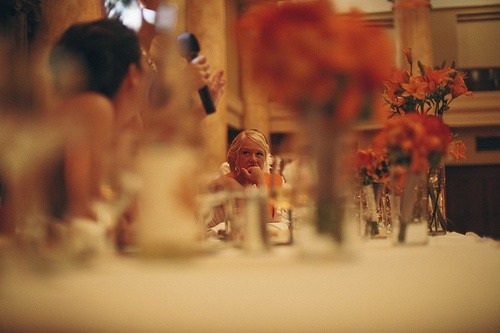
[424,164,447,236]
[374,190,393,239]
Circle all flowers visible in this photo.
[241,0,474,245]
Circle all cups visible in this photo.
[225,186,268,252]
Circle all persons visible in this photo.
[1,16,154,263]
[205,128,284,227]
[115,45,226,143]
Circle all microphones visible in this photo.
[177,32,217,115]
